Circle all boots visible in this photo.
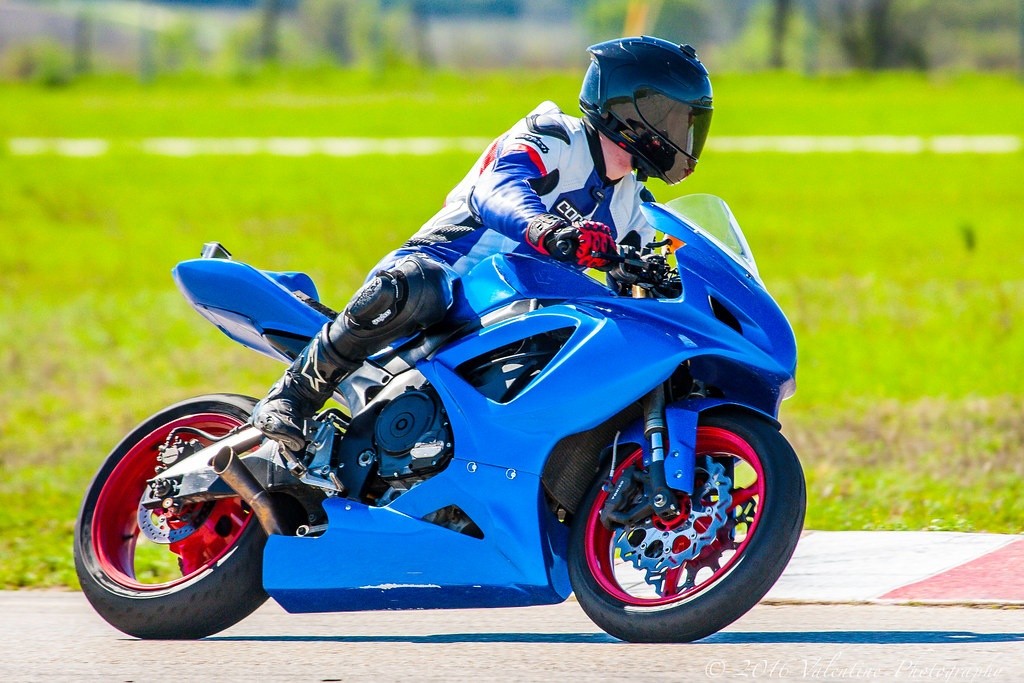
[253,321,363,451]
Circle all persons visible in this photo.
[247,35,713,451]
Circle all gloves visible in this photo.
[525,213,616,268]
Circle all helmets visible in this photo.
[578,36,713,186]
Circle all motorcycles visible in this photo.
[73,193,807,644]
